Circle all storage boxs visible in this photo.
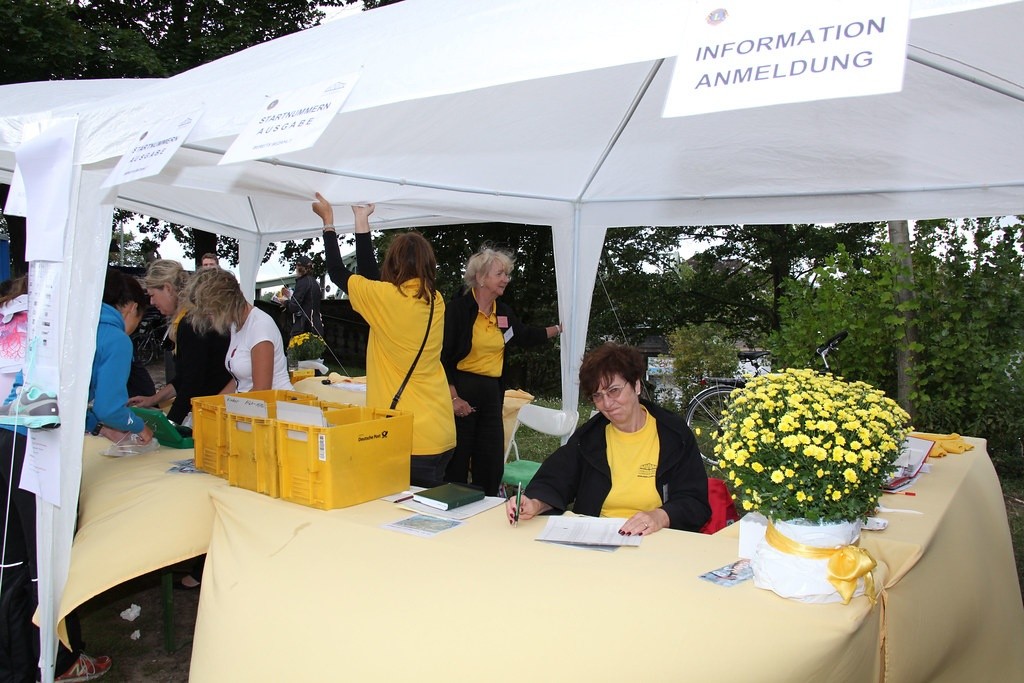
[186,390,415,510]
[288,370,315,383]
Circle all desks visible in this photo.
[296,373,534,457]
[33,431,1024,683]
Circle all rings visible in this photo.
[460,413,463,415]
[644,522,649,529]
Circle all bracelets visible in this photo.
[555,325,560,336]
[92,422,104,436]
[451,397,460,402]
[322,224,336,232]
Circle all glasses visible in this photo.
[587,380,629,403]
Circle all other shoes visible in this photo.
[181,575,199,588]
[37,653,112,683]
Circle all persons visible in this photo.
[312,192,458,486]
[435,246,562,497]
[506,343,713,536]
[0,251,324,683]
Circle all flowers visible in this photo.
[287,332,327,361]
[710,368,915,527]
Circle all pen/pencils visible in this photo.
[515,481,522,528]
[897,492,917,496]
[394,495,414,503]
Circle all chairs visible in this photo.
[501,404,581,490]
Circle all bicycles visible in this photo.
[129,317,172,366]
[685,331,849,467]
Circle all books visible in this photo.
[413,482,486,511]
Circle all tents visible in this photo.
[36,0,1024,682]
[0,76,165,216]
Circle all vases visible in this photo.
[298,358,323,368]
[773,517,860,550]
